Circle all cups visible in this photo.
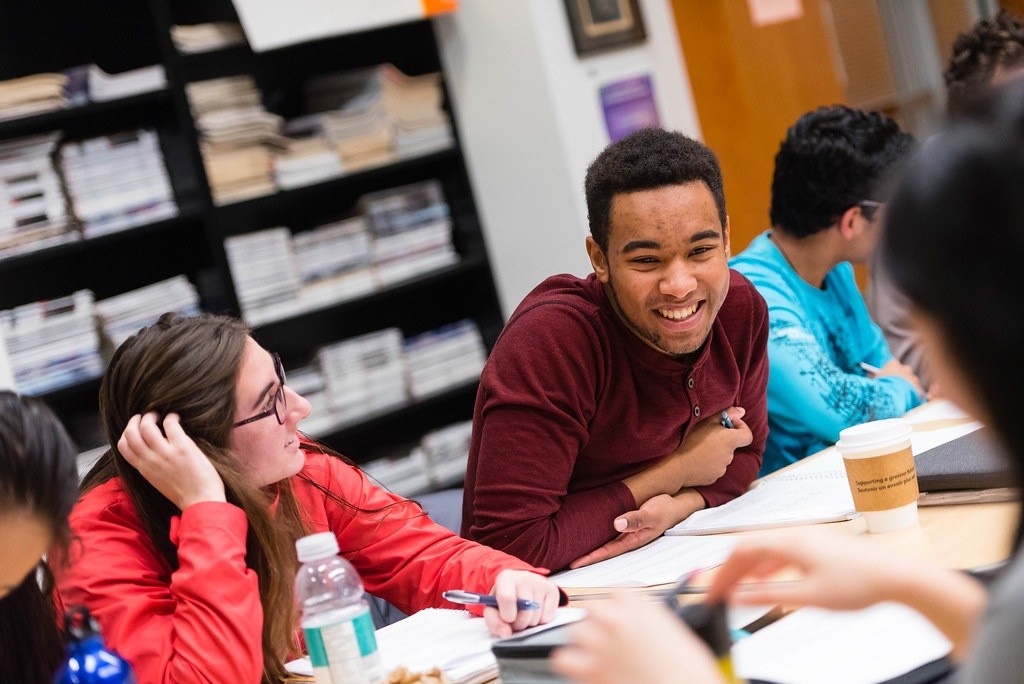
[835,418,920,534]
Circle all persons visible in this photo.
[726,104,925,478]
[45,311,571,684]
[0,390,138,683]
[866,11,1024,400]
[460,127,768,576]
[549,74,1024,684]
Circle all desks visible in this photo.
[284,397,1021,684]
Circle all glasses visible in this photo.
[230,352,287,428]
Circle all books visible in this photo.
[666,427,1016,536]
[280,606,584,683]
[0,21,488,496]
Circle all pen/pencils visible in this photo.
[442,589,542,611]
[722,411,736,430]
[856,361,882,375]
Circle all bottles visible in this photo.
[293,531,385,684]
[50,607,134,684]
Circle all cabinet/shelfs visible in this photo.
[0,0,505,500]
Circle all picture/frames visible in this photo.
[565,0,647,59]
